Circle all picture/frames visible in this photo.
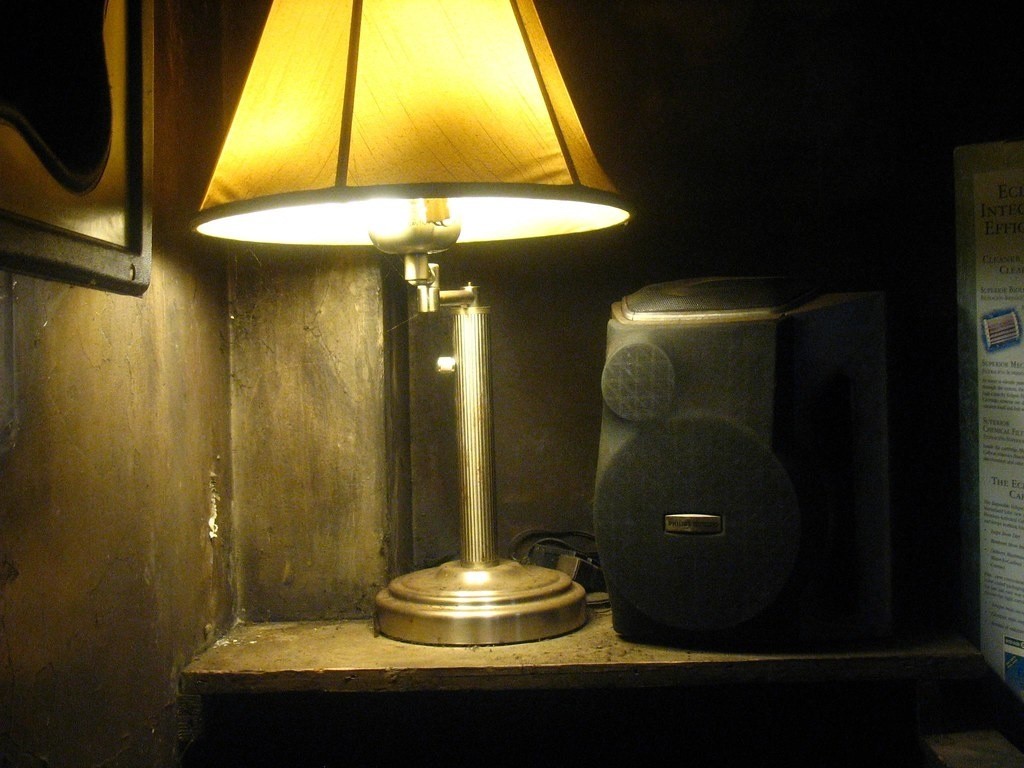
[0,0,154,295]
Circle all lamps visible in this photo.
[188,0,628,648]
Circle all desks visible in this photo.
[180,608,975,699]
